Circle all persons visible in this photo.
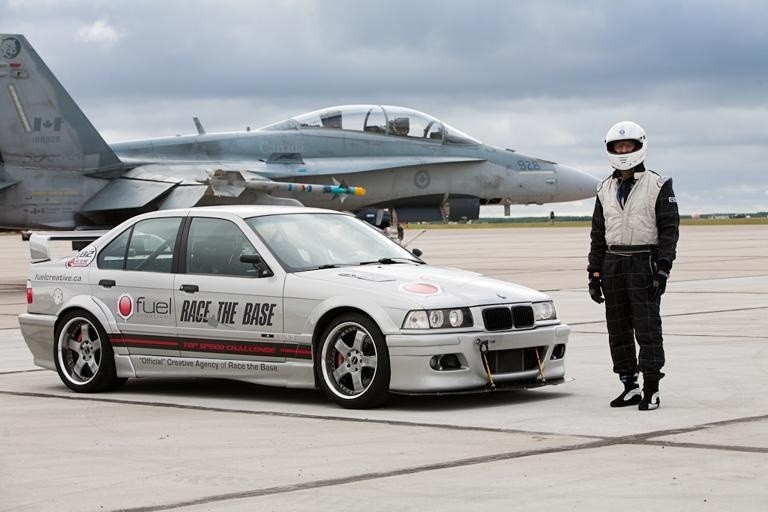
[585,119,679,411]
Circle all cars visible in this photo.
[19,204,571,409]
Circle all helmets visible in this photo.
[602,119,648,171]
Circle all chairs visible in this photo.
[191,238,230,274]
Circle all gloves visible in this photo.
[645,266,670,302]
[589,271,605,304]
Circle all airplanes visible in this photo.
[0,32,603,230]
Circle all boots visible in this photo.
[637,376,661,410]
[610,372,643,408]
